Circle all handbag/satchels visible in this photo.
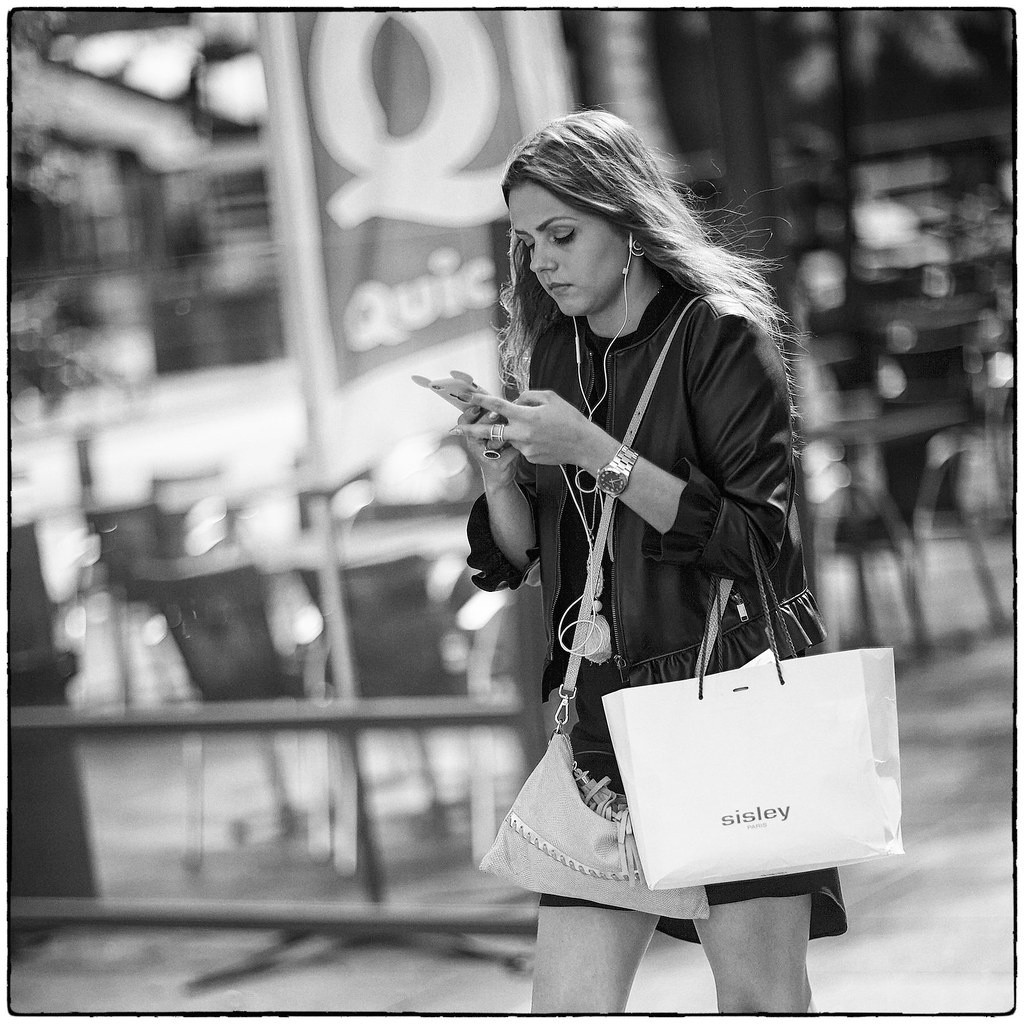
[602,518,911,890]
[478,736,712,922]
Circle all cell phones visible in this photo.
[411,371,508,428]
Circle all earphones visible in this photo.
[629,232,634,249]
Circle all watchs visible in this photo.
[596,444,639,499]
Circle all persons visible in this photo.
[449,109,848,1013]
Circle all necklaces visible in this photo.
[573,466,613,666]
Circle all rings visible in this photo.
[483,439,505,460]
[490,423,505,442]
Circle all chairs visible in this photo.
[810,397,1004,665]
[84,465,521,874]
